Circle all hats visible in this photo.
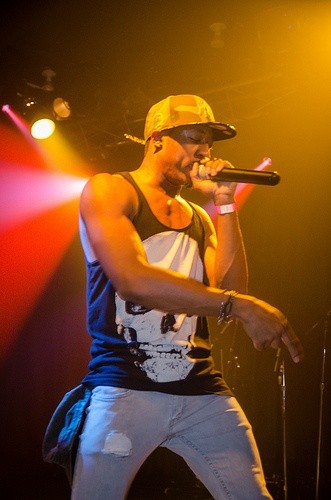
[144,95,238,142]
[42,383,93,486]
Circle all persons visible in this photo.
[72,94,301,500]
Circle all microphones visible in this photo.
[198,164,280,186]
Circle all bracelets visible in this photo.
[217,290,239,324]
[215,202,237,216]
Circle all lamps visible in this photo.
[23,95,56,140]
